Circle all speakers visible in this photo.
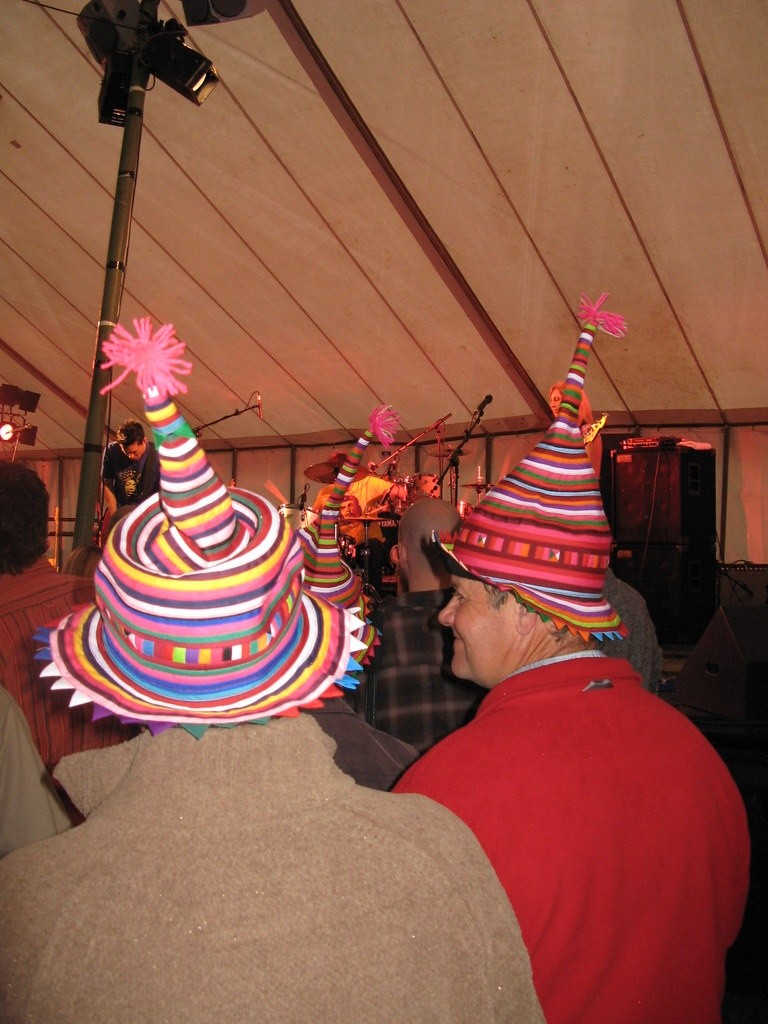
[610,446,768,724]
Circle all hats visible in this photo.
[444,292,629,643]
[295,404,402,626]
[34,316,368,740]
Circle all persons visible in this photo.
[0,316,547,1024]
[0,404,662,862]
[387,294,750,1024]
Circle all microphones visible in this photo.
[392,455,397,469]
[743,582,753,596]
[257,392,262,419]
[368,461,376,473]
[301,485,307,503]
[473,393,493,416]
[6,423,32,435]
